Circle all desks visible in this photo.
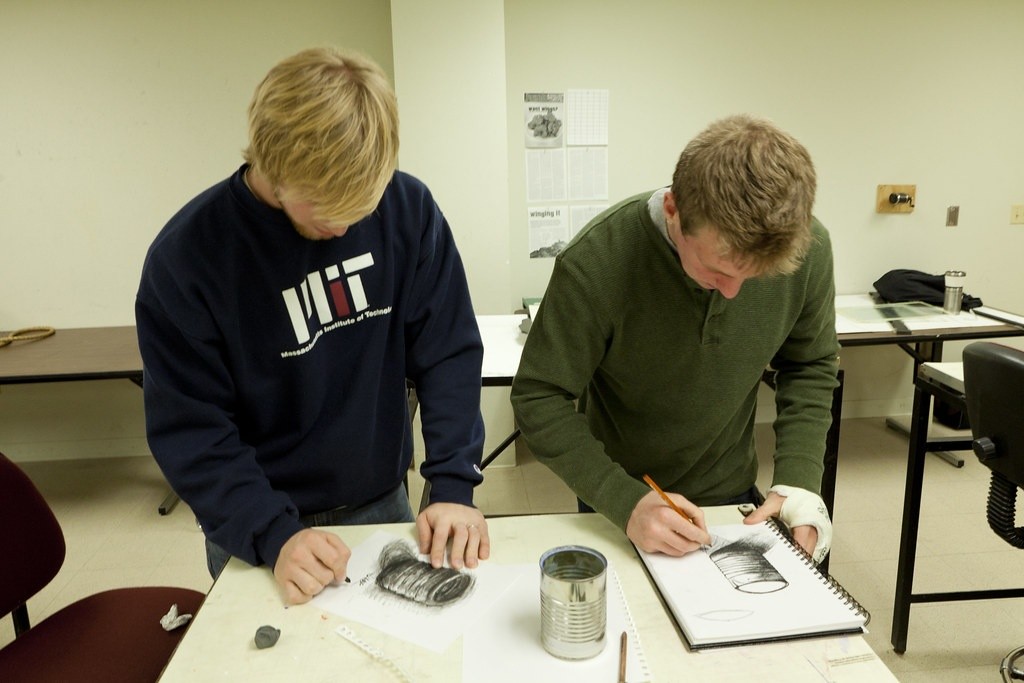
[0,329,182,515]
[407,314,532,471]
[890,358,1024,656]
[159,512,899,683]
[760,292,1024,523]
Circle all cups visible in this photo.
[943,271,966,315]
[539,545,607,660]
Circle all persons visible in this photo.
[133,45,492,616]
[503,113,840,571]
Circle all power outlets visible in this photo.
[1011,204,1024,225]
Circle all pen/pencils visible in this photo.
[619,631,628,682]
[641,473,712,547]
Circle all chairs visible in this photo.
[0,451,209,683]
[963,341,1024,682]
